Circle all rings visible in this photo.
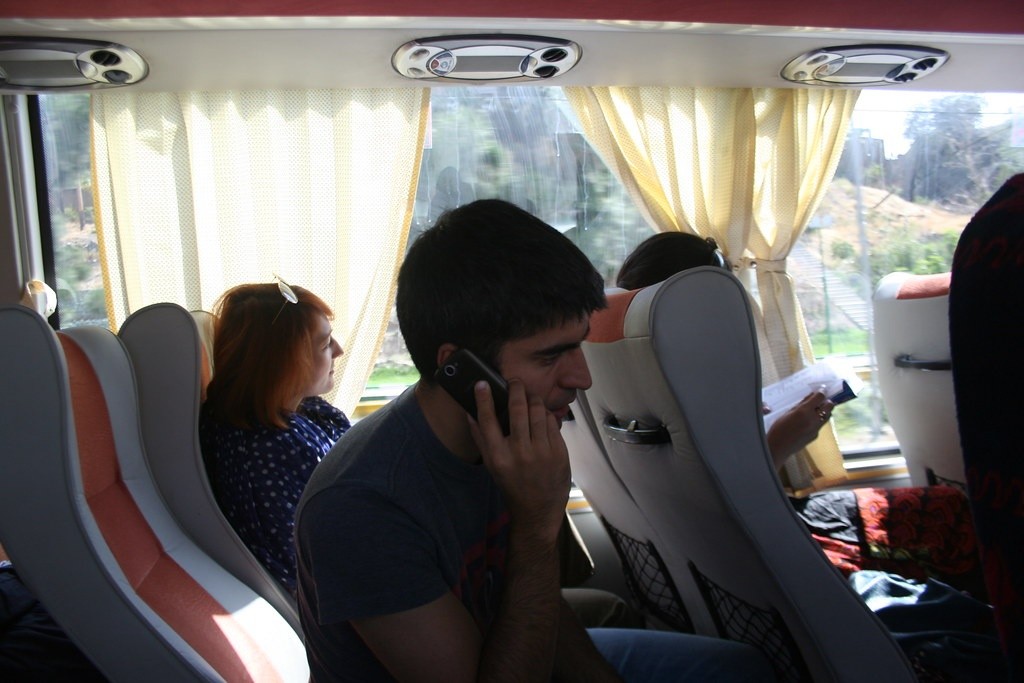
[819,409,827,420]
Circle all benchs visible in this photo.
[0,303,313,683]
[559,265,918,683]
[870,271,970,492]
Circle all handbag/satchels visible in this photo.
[847,570,996,658]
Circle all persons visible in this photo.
[605,229,986,597]
[289,198,790,683]
[198,283,635,632]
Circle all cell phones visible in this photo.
[433,349,522,436]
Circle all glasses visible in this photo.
[705,237,725,268]
[271,275,298,324]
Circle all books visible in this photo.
[762,352,863,433]
